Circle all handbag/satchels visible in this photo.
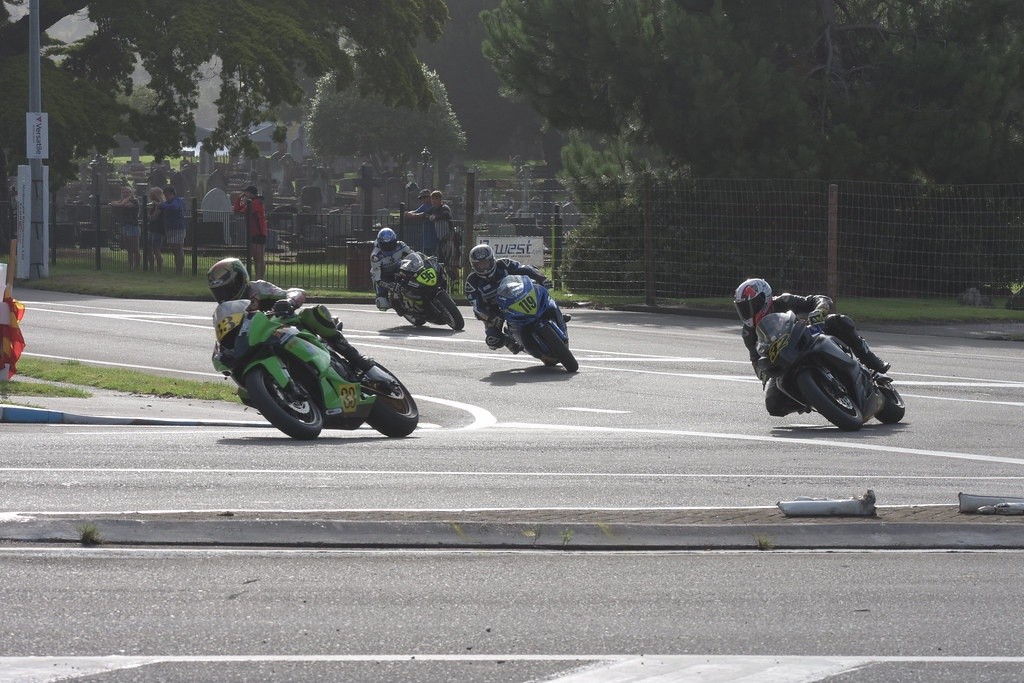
[253,234,266,244]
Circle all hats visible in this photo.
[242,186,258,194]
[430,190,443,197]
[417,188,431,199]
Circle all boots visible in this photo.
[562,314,571,322]
[329,332,361,369]
[854,339,891,373]
[392,300,404,317]
[499,335,519,354]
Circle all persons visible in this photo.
[110,185,186,276]
[461,243,571,352]
[730,279,890,418]
[371,229,437,320]
[205,258,375,379]
[404,187,452,257]
[233,186,268,281]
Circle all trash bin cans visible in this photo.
[346,241,374,291]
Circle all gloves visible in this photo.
[489,315,504,330]
[274,298,298,317]
[387,282,404,294]
[807,308,830,325]
[760,370,773,385]
[540,276,553,289]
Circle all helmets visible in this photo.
[734,278,772,328]
[206,258,251,306]
[469,244,497,278]
[376,227,397,256]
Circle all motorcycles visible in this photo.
[475,272,583,372]
[753,309,908,430]
[207,299,420,442]
[373,250,466,332]
